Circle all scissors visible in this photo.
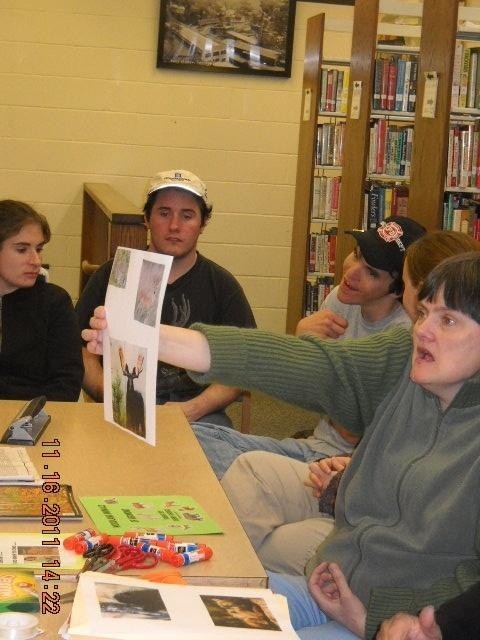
[108,550,159,574]
[76,544,114,580]
[96,545,140,573]
[105,547,145,573]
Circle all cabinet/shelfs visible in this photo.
[408,0,480,233]
[334,0,422,283]
[284,14,351,338]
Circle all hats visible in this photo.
[142,169,208,207]
[343,215,428,273]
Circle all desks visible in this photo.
[0,400,269,592]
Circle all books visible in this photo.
[301,62,352,315]
[359,47,418,231]
[439,38,479,245]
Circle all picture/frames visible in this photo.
[156,0,297,78]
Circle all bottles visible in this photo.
[63,526,213,568]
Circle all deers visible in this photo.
[119,348,145,435]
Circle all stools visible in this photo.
[220,390,253,436]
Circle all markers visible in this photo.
[157,541,206,554]
[75,533,111,555]
[125,531,173,541]
[109,536,156,546]
[64,528,96,550]
[137,543,174,562]
[171,547,214,568]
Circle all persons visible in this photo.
[373,571,480,640]
[0,199,85,402]
[190,218,424,478]
[83,252,479,640]
[77,170,261,427]
[216,233,479,572]
